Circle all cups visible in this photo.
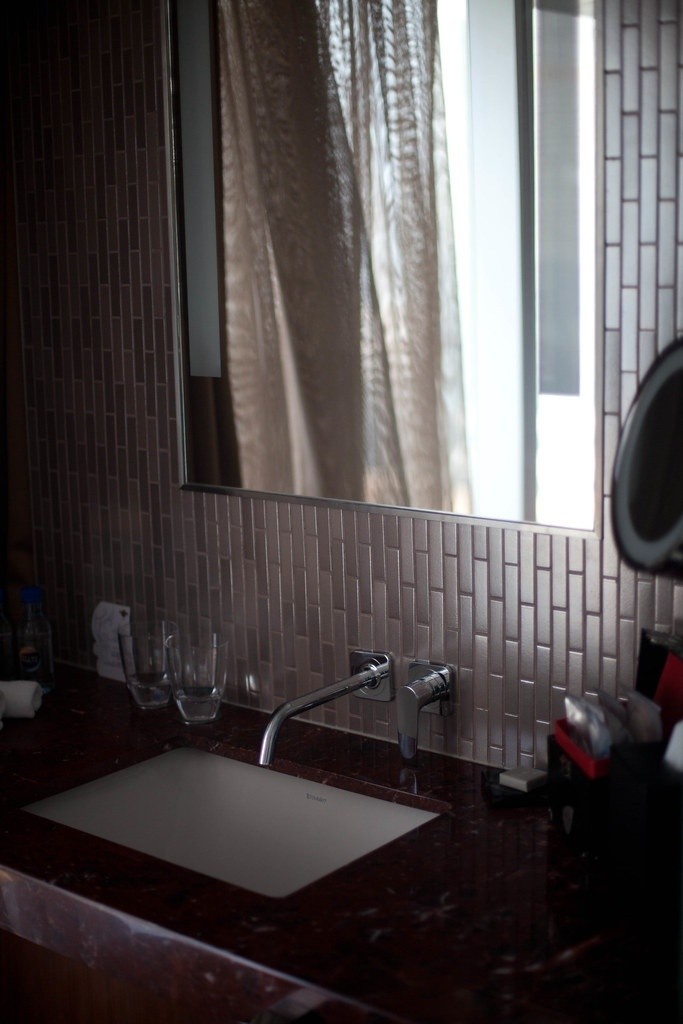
[163,633,229,721]
[117,621,179,711]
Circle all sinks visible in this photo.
[18,739,452,898]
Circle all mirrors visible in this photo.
[167,0,597,532]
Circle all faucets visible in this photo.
[258,649,395,768]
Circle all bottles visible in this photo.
[16,587,54,694]
